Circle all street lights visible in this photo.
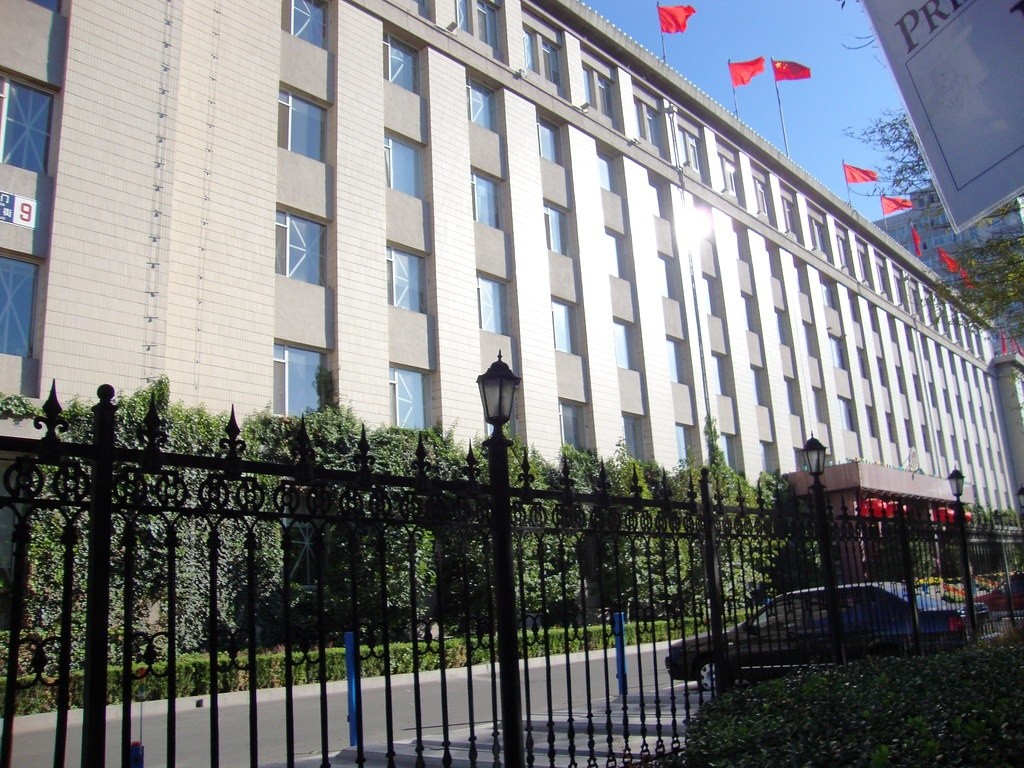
[804,430,844,668]
[477,350,526,768]
[947,466,978,644]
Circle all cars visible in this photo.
[973,571,1024,621]
[666,583,987,692]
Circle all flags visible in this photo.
[1000,329,1024,358]
[728,56,812,89]
[843,163,979,292]
[658,5,696,35]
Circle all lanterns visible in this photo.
[860,497,972,523]
